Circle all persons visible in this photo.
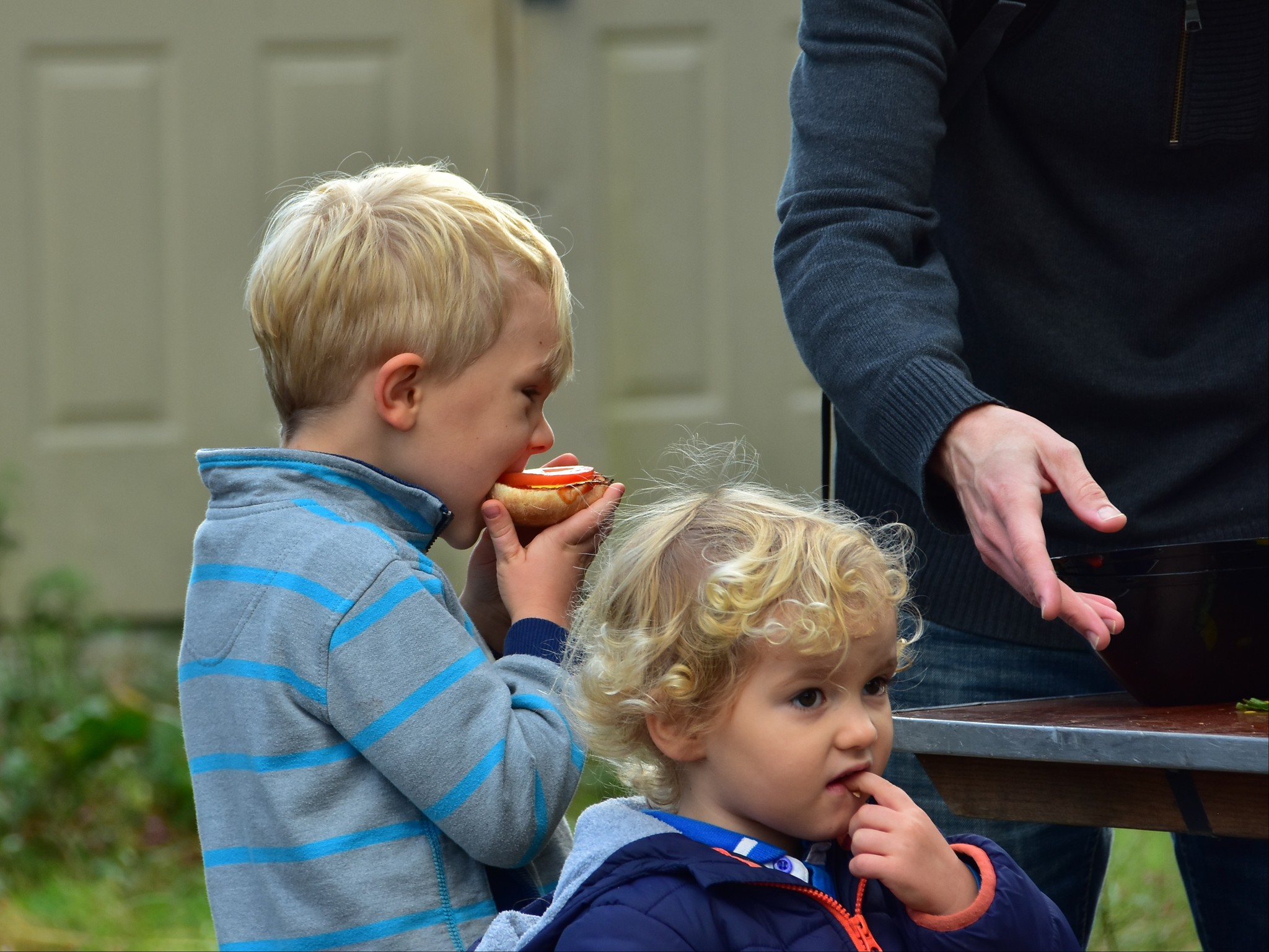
[767,0,1269,952]
[178,162,626,952]
[449,434,1087,952]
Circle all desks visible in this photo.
[863,690,1269,853]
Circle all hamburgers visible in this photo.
[487,465,610,525]
